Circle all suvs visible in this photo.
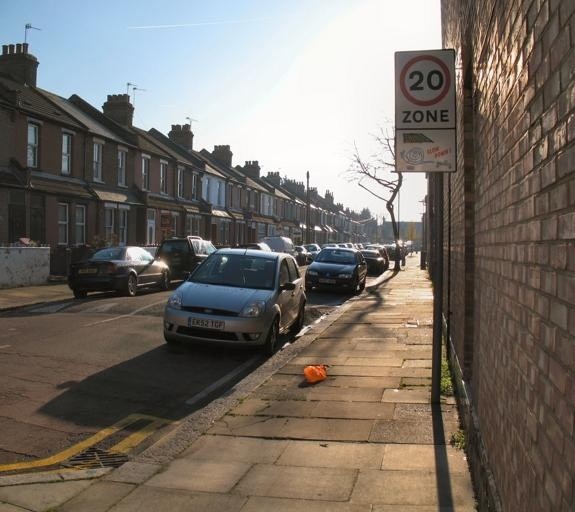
[156,236,217,281]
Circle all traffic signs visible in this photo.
[394,47,457,130]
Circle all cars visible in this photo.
[357,242,409,274]
[69,245,169,298]
[305,248,367,296]
[235,237,364,265]
[164,249,307,358]
